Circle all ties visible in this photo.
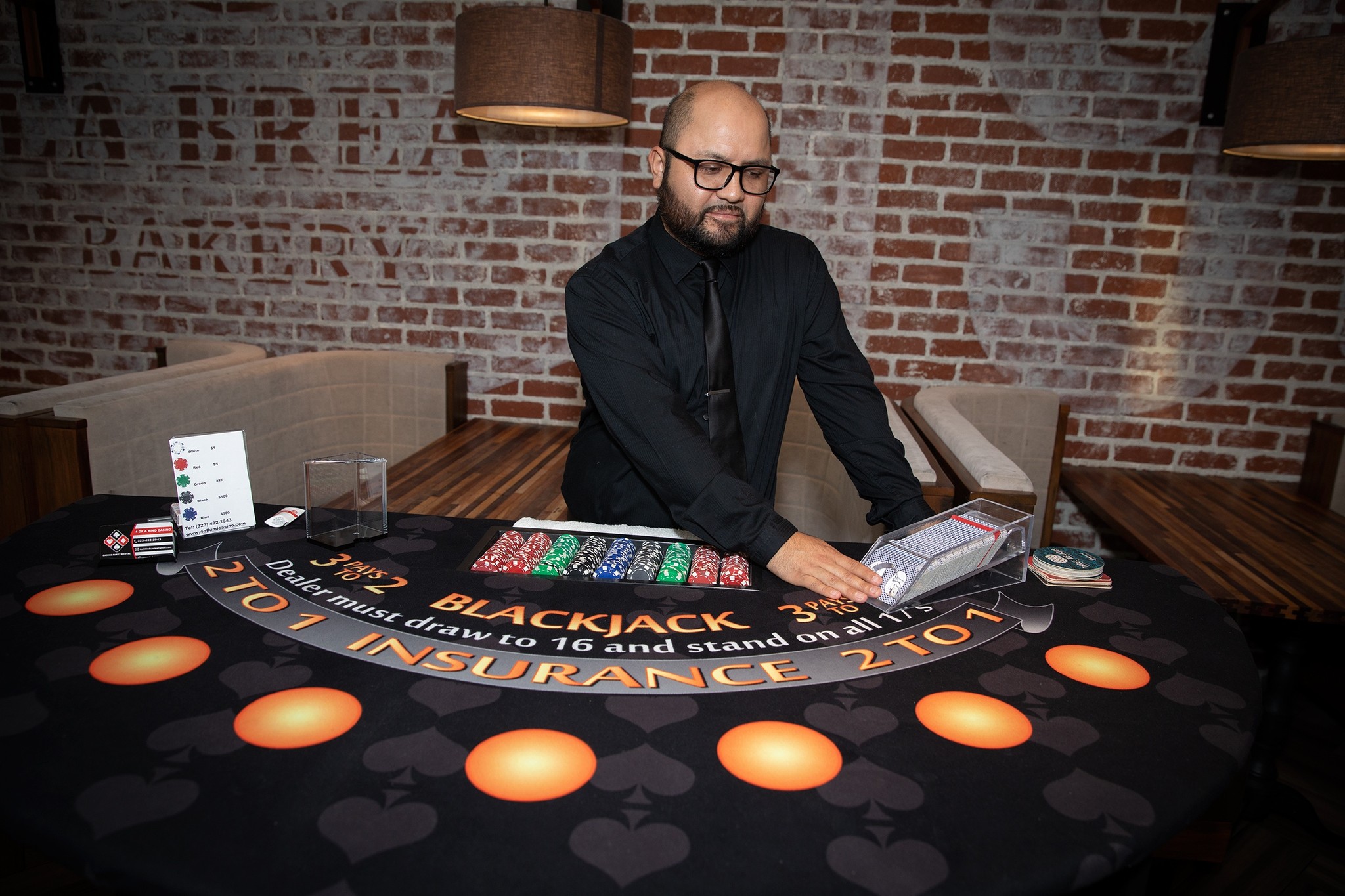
[697,256,748,485]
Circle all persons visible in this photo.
[562,81,938,604]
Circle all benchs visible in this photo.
[900,386,1070,548]
[775,378,953,544]
[1,340,265,537]
[26,350,468,516]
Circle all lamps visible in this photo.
[1197,0,1345,161]
[452,2,633,129]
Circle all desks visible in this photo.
[315,417,577,522]
[0,495,1345,896]
[1059,465,1344,896]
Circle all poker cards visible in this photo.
[859,511,1009,607]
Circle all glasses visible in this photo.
[661,146,780,195]
